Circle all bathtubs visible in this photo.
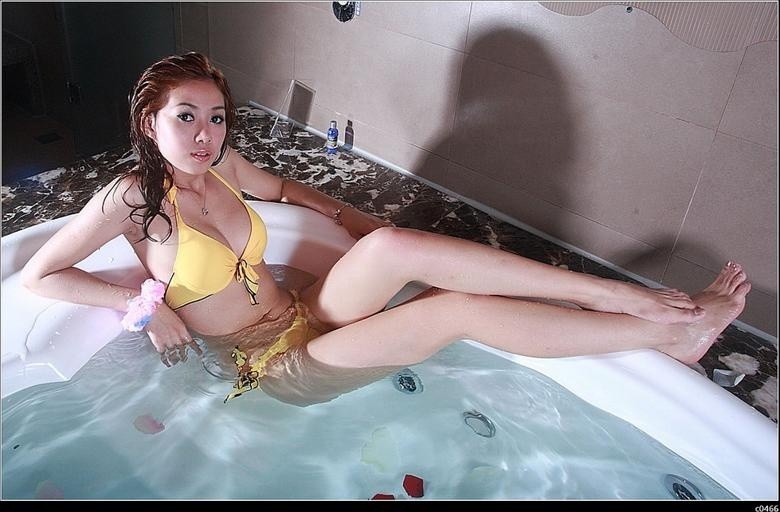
[1,199,775,502]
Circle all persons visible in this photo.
[19,50,755,408]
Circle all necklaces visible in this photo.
[178,182,211,216]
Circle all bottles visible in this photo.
[341,120,354,150]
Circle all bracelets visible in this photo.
[333,201,353,227]
[121,277,168,331]
